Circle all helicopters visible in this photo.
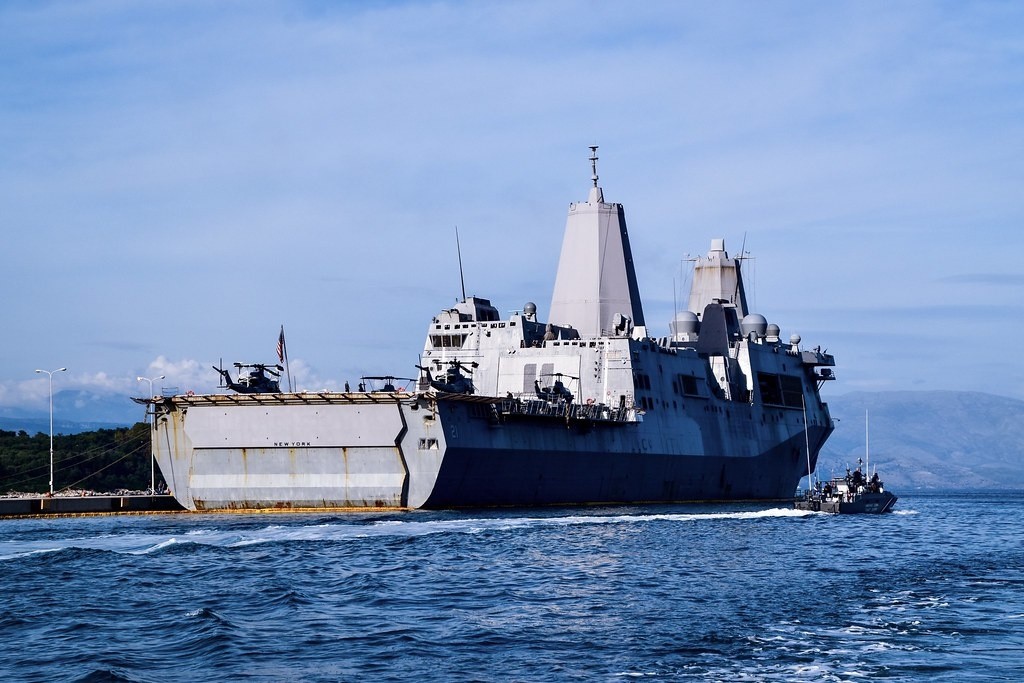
[212,357,284,393]
[360,375,419,392]
[415,356,479,395]
[534,373,579,403]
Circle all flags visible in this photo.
[276,332,284,364]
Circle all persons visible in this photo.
[871,473,879,493]
[823,482,832,498]
[813,481,821,495]
[853,468,862,484]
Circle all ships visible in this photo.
[129,146,840,512]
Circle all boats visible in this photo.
[892,510,920,516]
[793,394,898,513]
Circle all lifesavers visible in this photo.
[186,390,195,394]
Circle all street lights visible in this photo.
[137,376,165,495]
[35,367,66,494]
